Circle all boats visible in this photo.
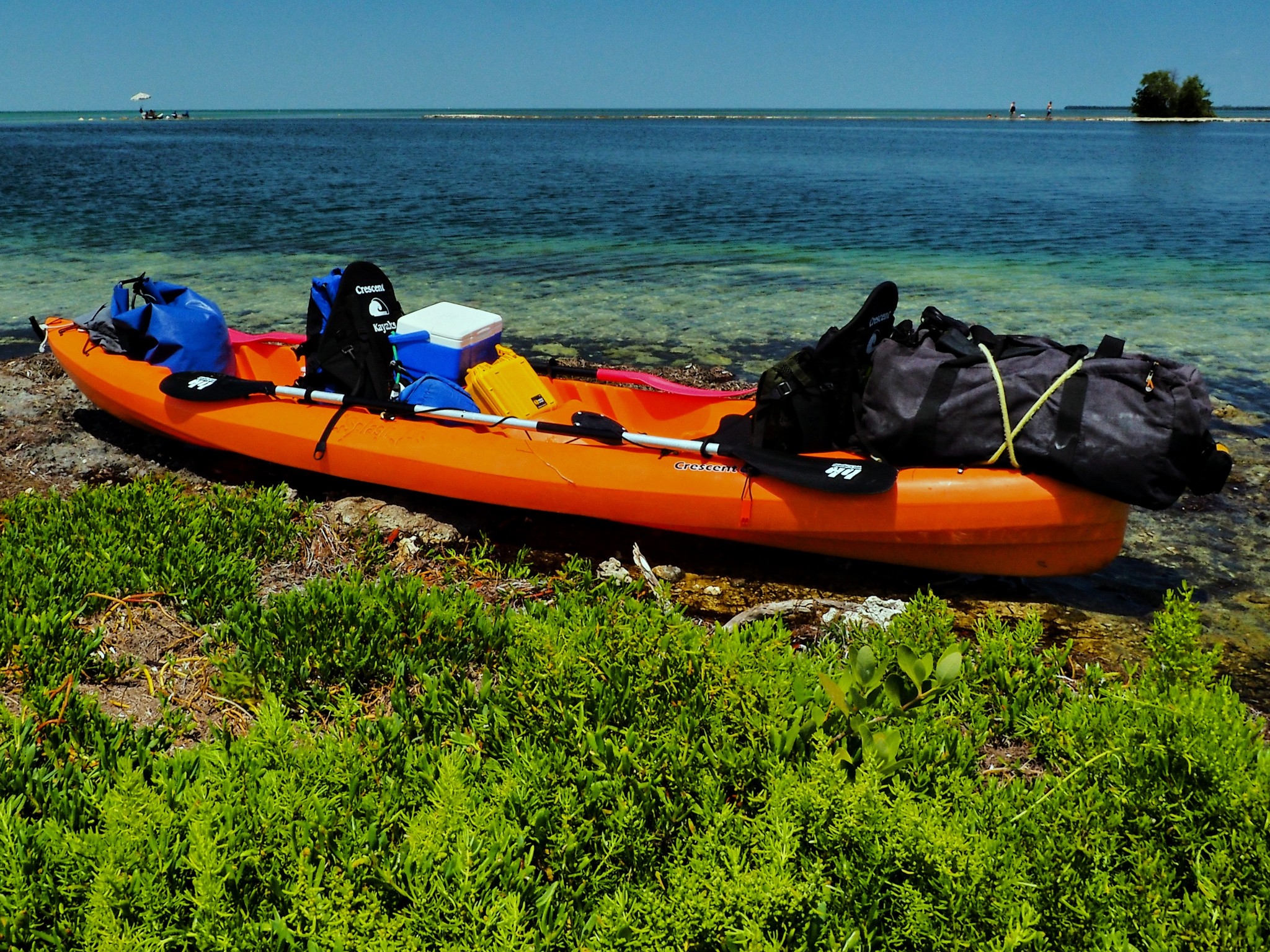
[26,313,1131,581]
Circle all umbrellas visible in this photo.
[131,92,151,112]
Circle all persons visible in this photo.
[172,111,178,118]
[1047,101,1052,118]
[182,111,189,117]
[1009,102,1016,118]
[140,108,164,119]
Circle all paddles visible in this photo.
[159,369,903,498]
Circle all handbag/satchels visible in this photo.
[854,306,1230,510]
[756,326,843,454]
